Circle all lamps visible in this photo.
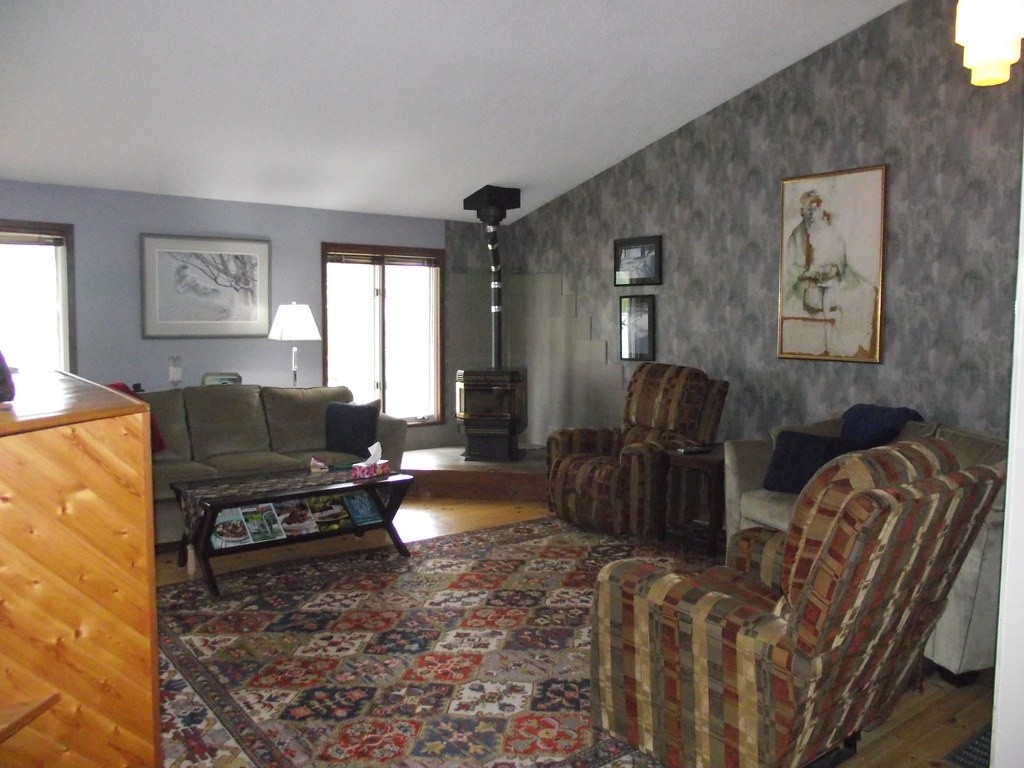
[267,301,323,386]
[953,0,1024,86]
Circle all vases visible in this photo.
[619,294,656,361]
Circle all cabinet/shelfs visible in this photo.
[0,369,167,768]
[662,441,724,556]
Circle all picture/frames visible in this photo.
[138,232,273,339]
[612,235,663,286]
[777,164,887,362]
[200,372,242,386]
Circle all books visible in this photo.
[210,488,385,552]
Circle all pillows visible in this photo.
[763,429,827,493]
[324,398,381,459]
[105,382,167,452]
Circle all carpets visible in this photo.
[153,511,726,767]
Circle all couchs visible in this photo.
[142,383,407,548]
[546,360,730,538]
[723,415,1008,686]
[588,435,1005,768]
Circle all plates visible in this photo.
[213,519,249,542]
[311,511,348,522]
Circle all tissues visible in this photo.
[351,441,391,479]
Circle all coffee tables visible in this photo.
[173,464,416,596]
